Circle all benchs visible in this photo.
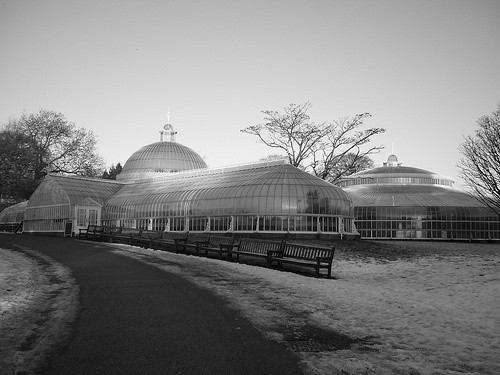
[77,224,337,278]
